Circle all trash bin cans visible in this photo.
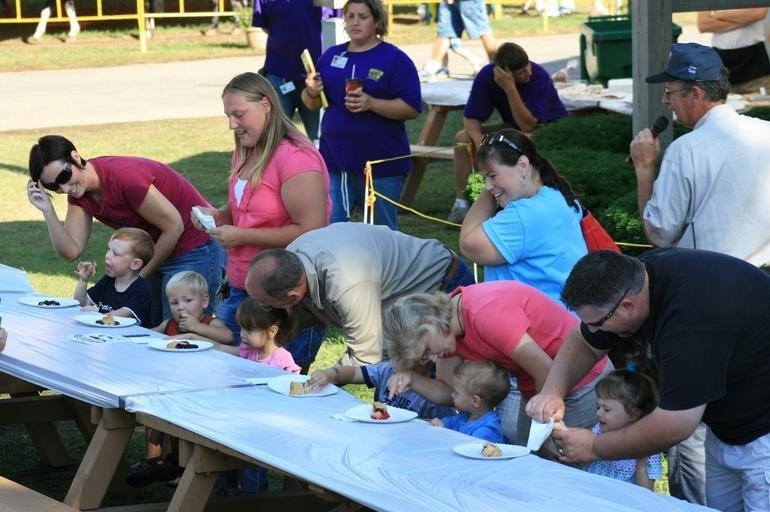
[579,12,683,88]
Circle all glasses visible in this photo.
[580,285,630,329]
[660,84,698,101]
[40,162,73,192]
[479,132,528,158]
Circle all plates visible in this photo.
[450,441,532,460]
[73,314,137,328]
[266,376,339,398]
[17,295,81,310]
[344,403,420,424]
[147,339,214,353]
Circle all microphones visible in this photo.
[624,116,668,165]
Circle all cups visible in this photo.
[344,78,363,111]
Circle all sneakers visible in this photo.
[445,198,470,230]
[125,455,174,488]
[415,60,451,83]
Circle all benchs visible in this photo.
[0,475,84,512]
[408,144,457,167]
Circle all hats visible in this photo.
[642,43,728,84]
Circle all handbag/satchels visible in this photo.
[580,198,626,258]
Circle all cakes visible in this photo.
[290,382,304,394]
[371,402,390,419]
[167,340,198,349]
[482,443,501,457]
[96,313,120,324]
